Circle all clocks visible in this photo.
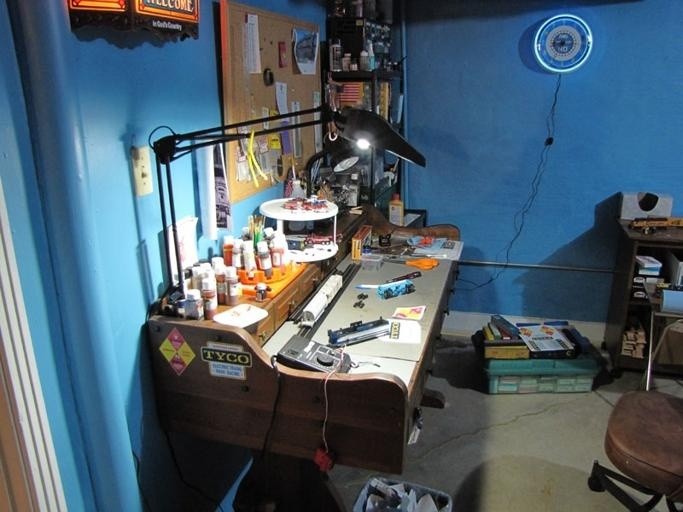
[531,14,594,75]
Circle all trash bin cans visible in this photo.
[353,475,453,512]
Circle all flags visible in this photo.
[336,83,361,102]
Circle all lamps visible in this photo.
[146,103,428,297]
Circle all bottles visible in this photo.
[330,39,375,69]
[387,194,403,227]
[185,229,284,322]
[289,179,305,230]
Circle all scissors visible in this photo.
[389,258,439,270]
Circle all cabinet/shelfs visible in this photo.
[323,1,409,218]
[145,207,462,475]
[600,219,683,384]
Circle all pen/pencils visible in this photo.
[354,284,379,289]
[248,214,266,249]
[384,272,421,284]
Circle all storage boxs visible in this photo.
[470,323,605,395]
